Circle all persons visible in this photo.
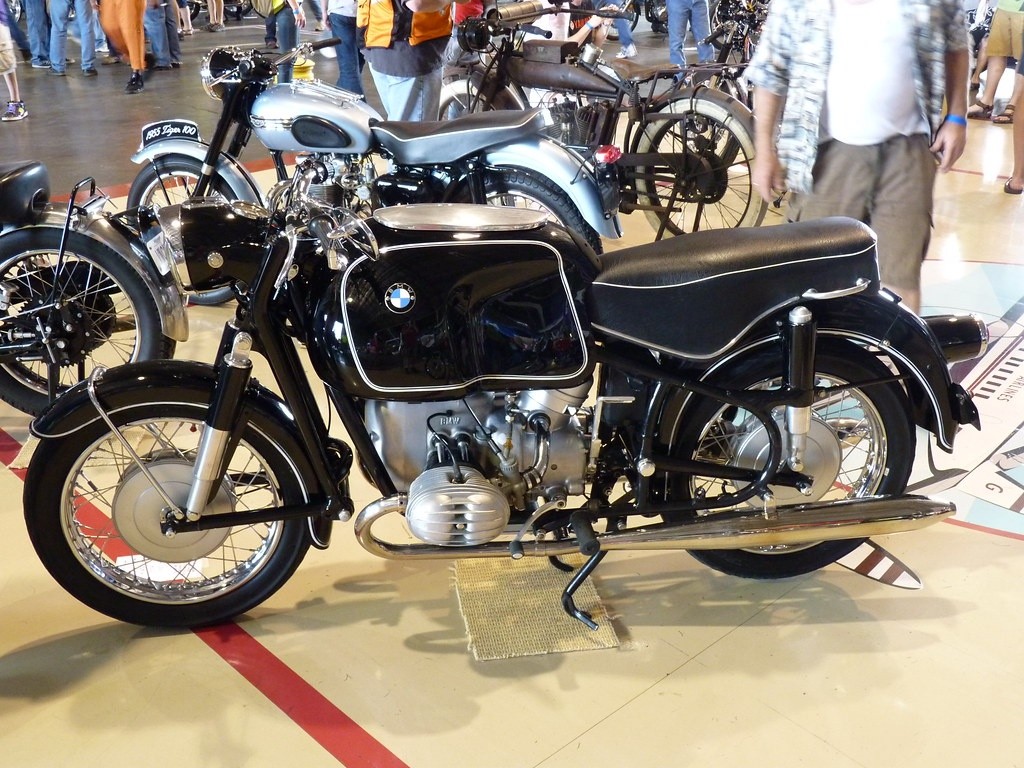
[199,0,226,33]
[522,0,638,61]
[968,0,1024,194]
[356,0,471,124]
[264,0,327,84]
[742,0,971,383]
[320,0,368,104]
[442,0,496,122]
[666,0,715,88]
[0,0,29,121]
[6,0,194,94]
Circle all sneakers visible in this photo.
[2,100,28,121]
[615,44,638,58]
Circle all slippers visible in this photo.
[1005,178,1023,194]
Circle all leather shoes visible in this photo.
[125,71,144,94]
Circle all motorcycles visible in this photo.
[437,0,768,243]
[4,0,253,26]
[0,161,191,418]
[126,38,624,306]
[22,151,990,632]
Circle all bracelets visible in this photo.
[293,9,302,15]
[945,113,968,127]
[587,22,594,30]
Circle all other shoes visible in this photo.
[46,66,66,76]
[143,54,154,70]
[170,62,181,67]
[211,23,225,32]
[84,68,98,77]
[154,64,172,70]
[95,43,109,53]
[31,61,51,68]
[200,23,213,32]
[314,22,326,31]
[177,32,184,41]
[101,54,121,65]
[266,40,279,49]
[65,58,75,64]
[183,27,194,35]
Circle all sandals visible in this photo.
[994,104,1015,123]
[967,101,994,119]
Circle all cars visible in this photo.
[594,0,769,63]
